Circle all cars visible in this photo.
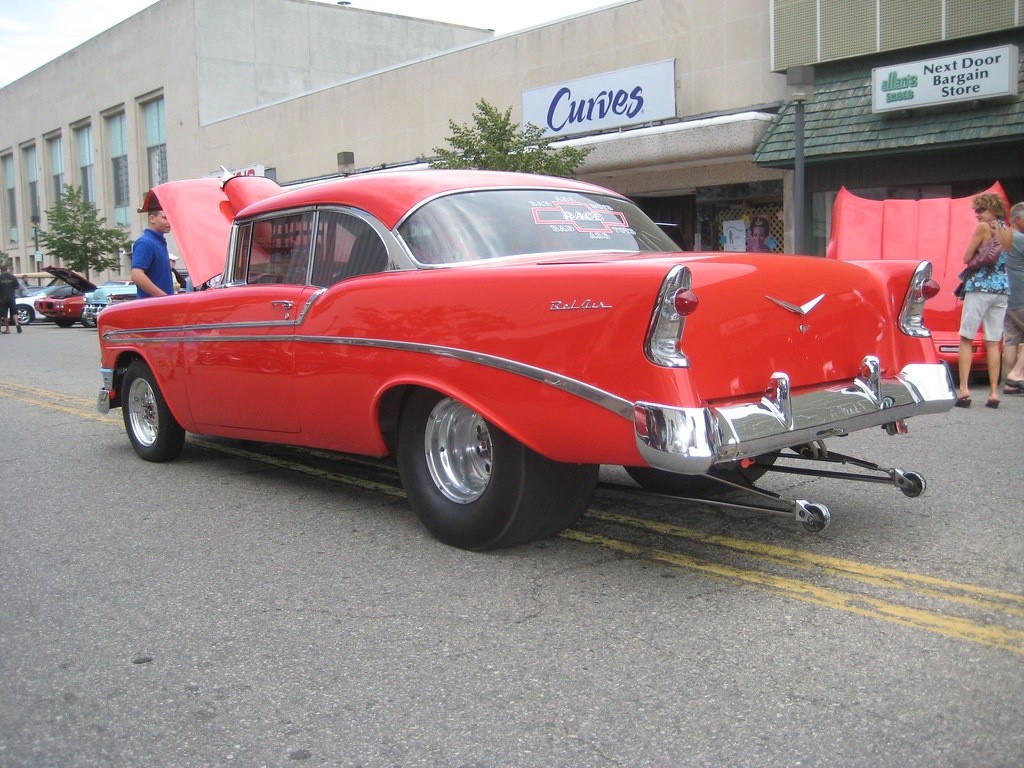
[33,265,134,328]
[8,286,59,325]
[820,181,1011,388]
[96,162,960,555]
[81,284,137,326]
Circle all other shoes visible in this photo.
[2,331,10,334]
[17,325,22,333]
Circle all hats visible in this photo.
[750,216,769,229]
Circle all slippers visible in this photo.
[956,399,971,407]
[1003,388,1024,393]
[1005,380,1024,390]
[986,399,999,408]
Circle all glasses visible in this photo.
[975,207,987,214]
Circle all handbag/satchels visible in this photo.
[968,221,1002,270]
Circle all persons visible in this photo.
[955,193,1013,409]
[169,254,181,294]
[729,230,733,244]
[131,211,174,299]
[1003,202,1024,394]
[750,217,770,251]
[19,275,30,287]
[0,265,22,334]
